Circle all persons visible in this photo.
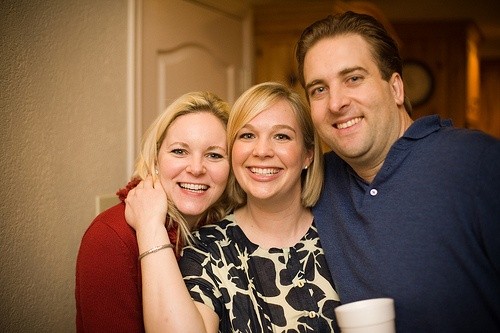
[124,81,344,333]
[295,11,500,333]
[74,90,245,333]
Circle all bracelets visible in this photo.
[138,244,175,260]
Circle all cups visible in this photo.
[334,297,395,333]
